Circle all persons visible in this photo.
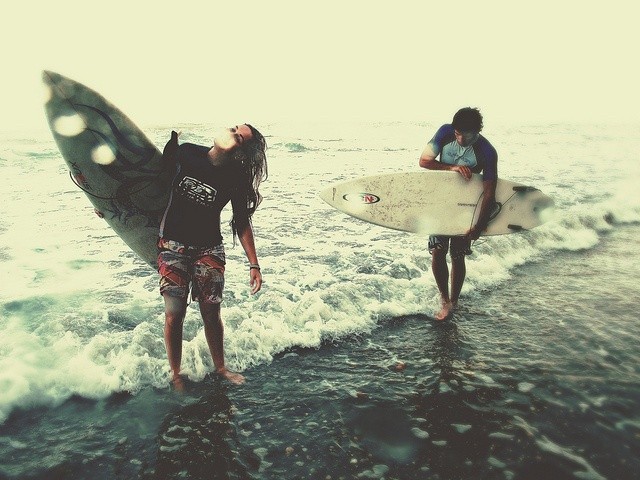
[419,107,498,321]
[93,123,268,395]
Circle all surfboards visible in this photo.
[319,170,555,237]
[41,69,163,272]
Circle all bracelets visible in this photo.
[474,222,483,232]
[250,263,261,270]
